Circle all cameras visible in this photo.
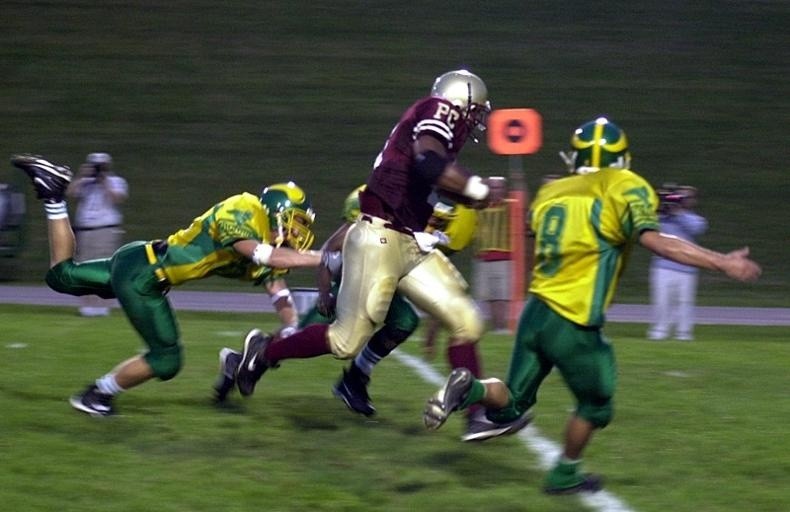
[87,162,108,177]
[655,181,689,207]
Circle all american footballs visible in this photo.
[462,176,510,210]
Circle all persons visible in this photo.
[477,174,515,336]
[9,152,346,418]
[66,152,129,320]
[648,184,711,342]
[237,69,536,443]
[425,119,760,493]
[211,181,476,416]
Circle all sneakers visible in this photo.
[233,327,277,397]
[331,366,377,418]
[648,330,667,342]
[211,347,244,407]
[95,308,113,318]
[79,308,95,317]
[69,383,116,418]
[10,152,74,204]
[460,406,535,443]
[423,368,473,434]
[542,473,606,496]
[673,333,694,341]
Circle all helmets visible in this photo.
[432,72,492,144]
[570,119,631,175]
[258,182,316,253]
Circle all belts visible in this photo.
[72,224,124,234]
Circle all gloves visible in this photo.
[319,248,340,275]
[463,174,507,203]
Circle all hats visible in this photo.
[86,153,112,163]
